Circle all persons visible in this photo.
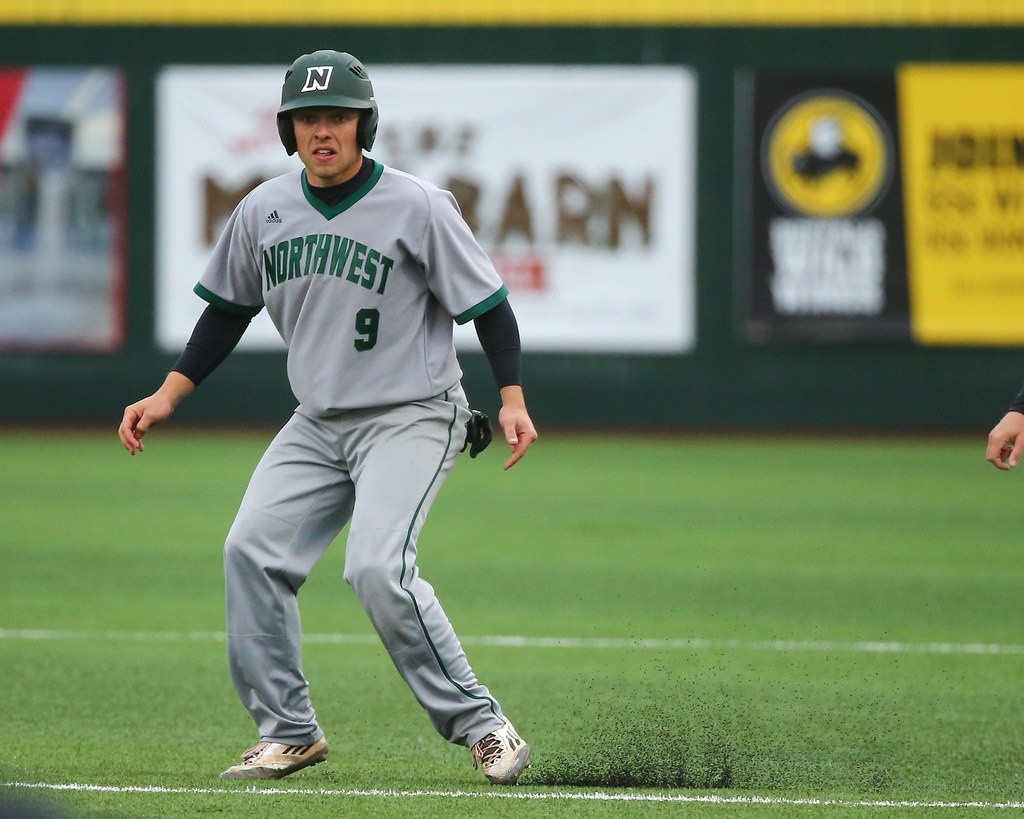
[987,387,1024,470]
[118,51,539,786]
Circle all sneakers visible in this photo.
[470,718,530,785]
[220,735,328,779]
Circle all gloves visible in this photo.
[460,410,493,458]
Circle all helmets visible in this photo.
[276,49,378,156]
